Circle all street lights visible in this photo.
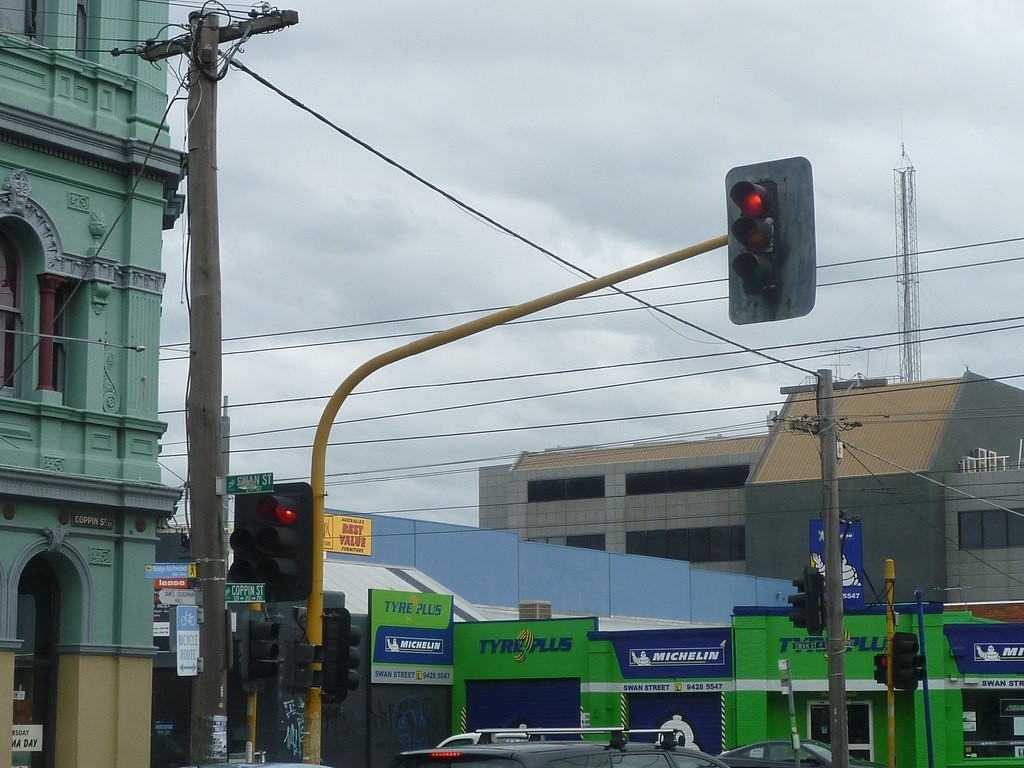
[714,739,889,768]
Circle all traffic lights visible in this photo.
[723,156,816,325]
[873,631,927,691]
[229,481,314,604]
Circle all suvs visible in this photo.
[386,727,732,768]
